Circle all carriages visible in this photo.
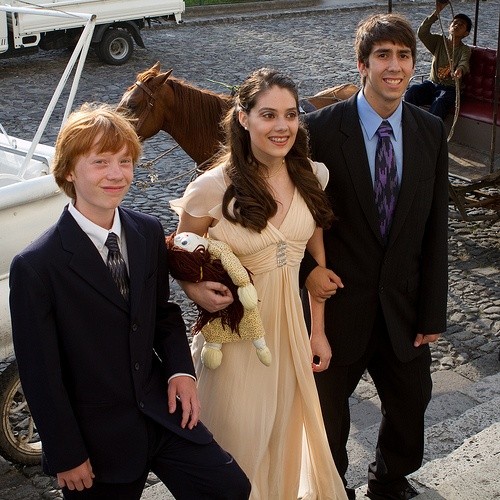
[119,0,500,222]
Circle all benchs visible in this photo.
[406,45,500,128]
[0,132,74,282]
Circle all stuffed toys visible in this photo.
[163,232,273,370]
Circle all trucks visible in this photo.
[0,0,186,65]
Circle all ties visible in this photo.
[104,232,130,306]
[373,120,399,238]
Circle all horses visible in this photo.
[114,62,361,181]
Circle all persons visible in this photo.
[405,0,473,142]
[167,68,332,500]
[299,14,449,500]
[8,100,252,500]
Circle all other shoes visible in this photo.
[367,482,421,500]
